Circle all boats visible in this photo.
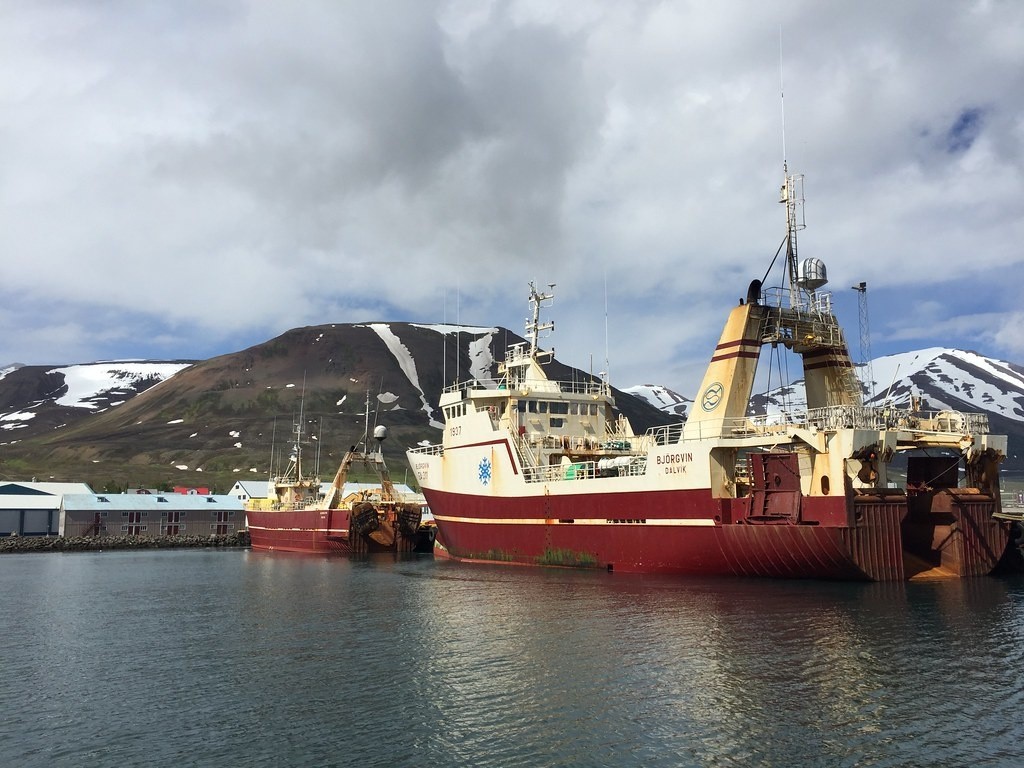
[243,369,441,554]
[406,7,1014,584]
